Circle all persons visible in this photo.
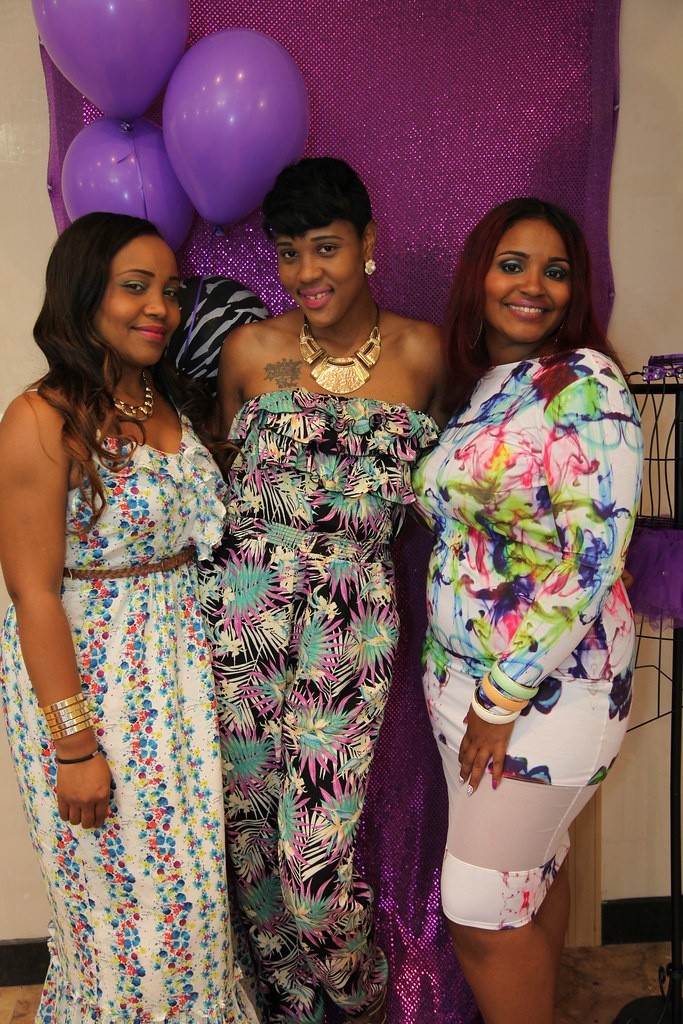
[198,147,455,1024]
[403,189,646,1024]
[1,203,264,1024]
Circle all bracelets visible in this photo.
[471,686,524,725]
[52,743,104,765]
[480,672,531,714]
[491,659,538,702]
[41,693,93,741]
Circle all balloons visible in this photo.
[59,120,196,271]
[29,0,195,138]
[163,25,310,240]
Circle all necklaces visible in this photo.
[106,366,157,423]
[298,298,382,396]
